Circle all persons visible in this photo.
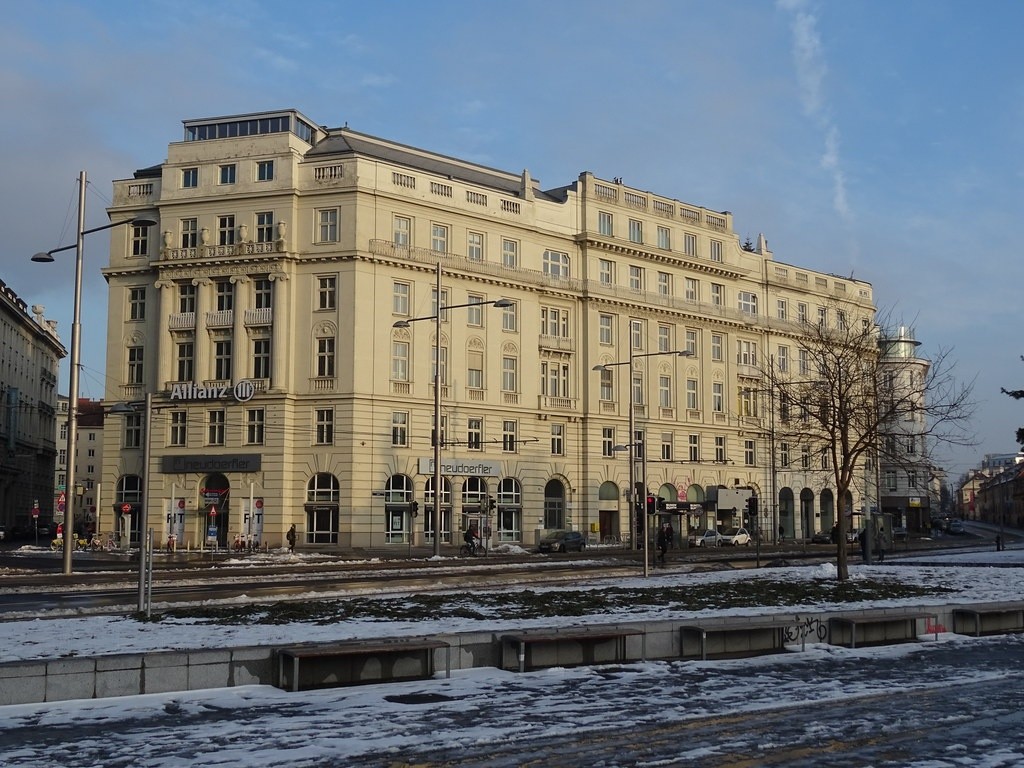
[925,517,945,536]
[287,524,296,553]
[995,534,1005,551]
[858,528,866,560]
[657,527,666,563]
[778,524,784,542]
[665,524,673,550]
[464,525,480,552]
[877,527,886,560]
[828,521,838,544]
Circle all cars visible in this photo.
[931,512,966,535]
[812,526,908,544]
[687,528,752,547]
[538,530,585,553]
[0,523,52,541]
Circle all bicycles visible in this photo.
[233,534,259,553]
[50,538,116,552]
[460,538,486,557]
[167,534,178,552]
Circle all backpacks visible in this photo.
[287,531,293,540]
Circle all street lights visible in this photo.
[591,351,694,551]
[393,299,512,555]
[31,215,157,574]
[738,381,828,546]
[611,439,648,578]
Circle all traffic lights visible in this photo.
[647,497,656,515]
[656,495,665,510]
[745,497,758,516]
[410,501,418,518]
[489,499,496,510]
[478,499,485,511]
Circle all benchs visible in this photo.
[828,613,938,649]
[501,628,647,673]
[953,608,1024,636]
[278,640,450,692]
[680,621,806,660]
